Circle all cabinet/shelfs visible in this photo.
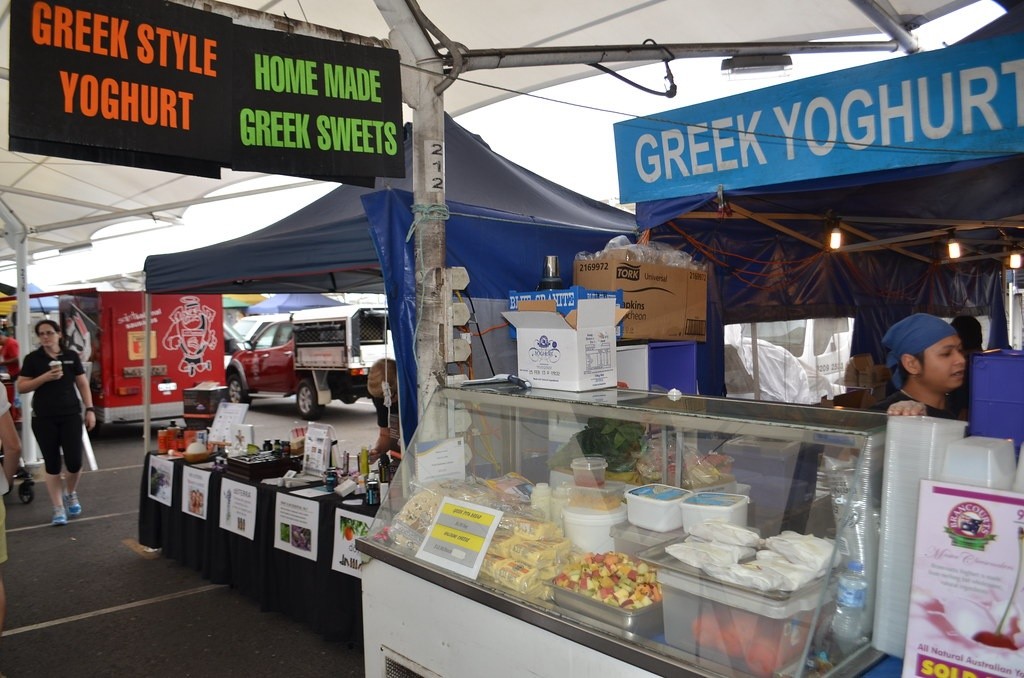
[617,342,699,463]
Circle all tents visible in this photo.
[143,112,1024,461]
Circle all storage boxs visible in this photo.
[183,385,228,430]
[822,389,878,410]
[334,472,367,498]
[499,248,709,391]
[226,452,302,480]
[655,566,837,677]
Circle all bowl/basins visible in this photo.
[871,412,1024,658]
[624,484,692,533]
[568,480,626,511]
[570,456,609,489]
[680,491,750,532]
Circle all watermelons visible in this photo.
[552,554,640,601]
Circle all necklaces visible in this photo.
[900,389,913,398]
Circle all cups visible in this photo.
[50,363,62,370]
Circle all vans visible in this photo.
[0,287,225,426]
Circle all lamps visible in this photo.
[719,54,795,76]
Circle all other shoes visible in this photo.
[63,489,81,516]
[52,508,68,525]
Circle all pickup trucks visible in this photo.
[223,303,396,421]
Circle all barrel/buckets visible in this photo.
[561,502,628,554]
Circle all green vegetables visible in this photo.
[544,414,650,473]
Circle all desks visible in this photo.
[139,450,382,644]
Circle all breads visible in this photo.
[391,480,567,596]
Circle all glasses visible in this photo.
[39,330,56,336]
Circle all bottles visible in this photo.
[365,454,390,505]
[829,561,868,637]
[325,467,339,492]
[273,439,281,451]
[530,482,550,522]
[158,420,196,453]
[539,255,563,291]
[551,482,571,528]
[263,439,272,451]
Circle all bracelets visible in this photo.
[86,407,95,412]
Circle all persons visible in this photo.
[1,330,24,638]
[14,320,96,525]
[865,313,984,508]
[367,358,401,462]
